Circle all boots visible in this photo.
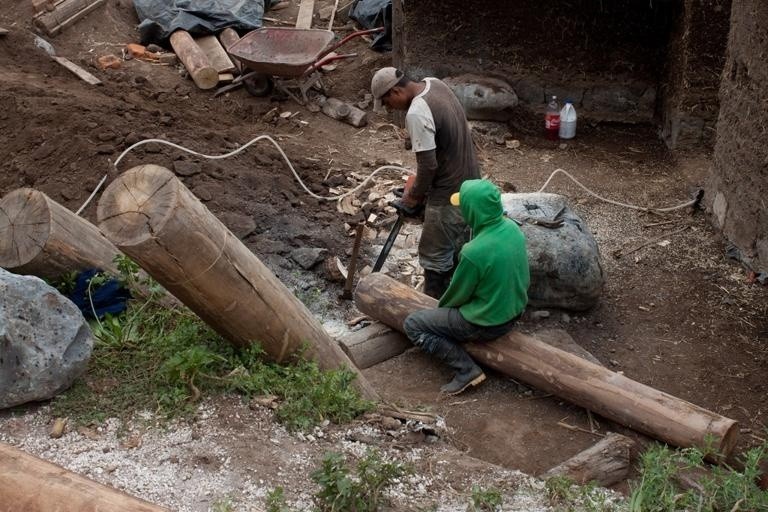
[432,336,486,396]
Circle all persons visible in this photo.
[402,177,533,396]
[370,65,482,298]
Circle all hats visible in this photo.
[372,66,405,114]
[450,192,460,206]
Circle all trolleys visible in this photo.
[226,26,384,106]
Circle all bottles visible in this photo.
[543,95,560,139]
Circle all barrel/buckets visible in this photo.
[542,94,560,148]
